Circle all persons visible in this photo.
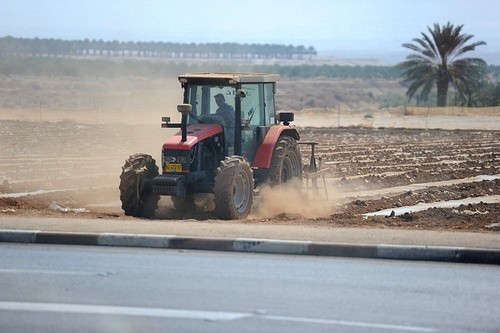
[213,93,234,126]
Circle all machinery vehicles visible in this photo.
[118,73,330,221]
